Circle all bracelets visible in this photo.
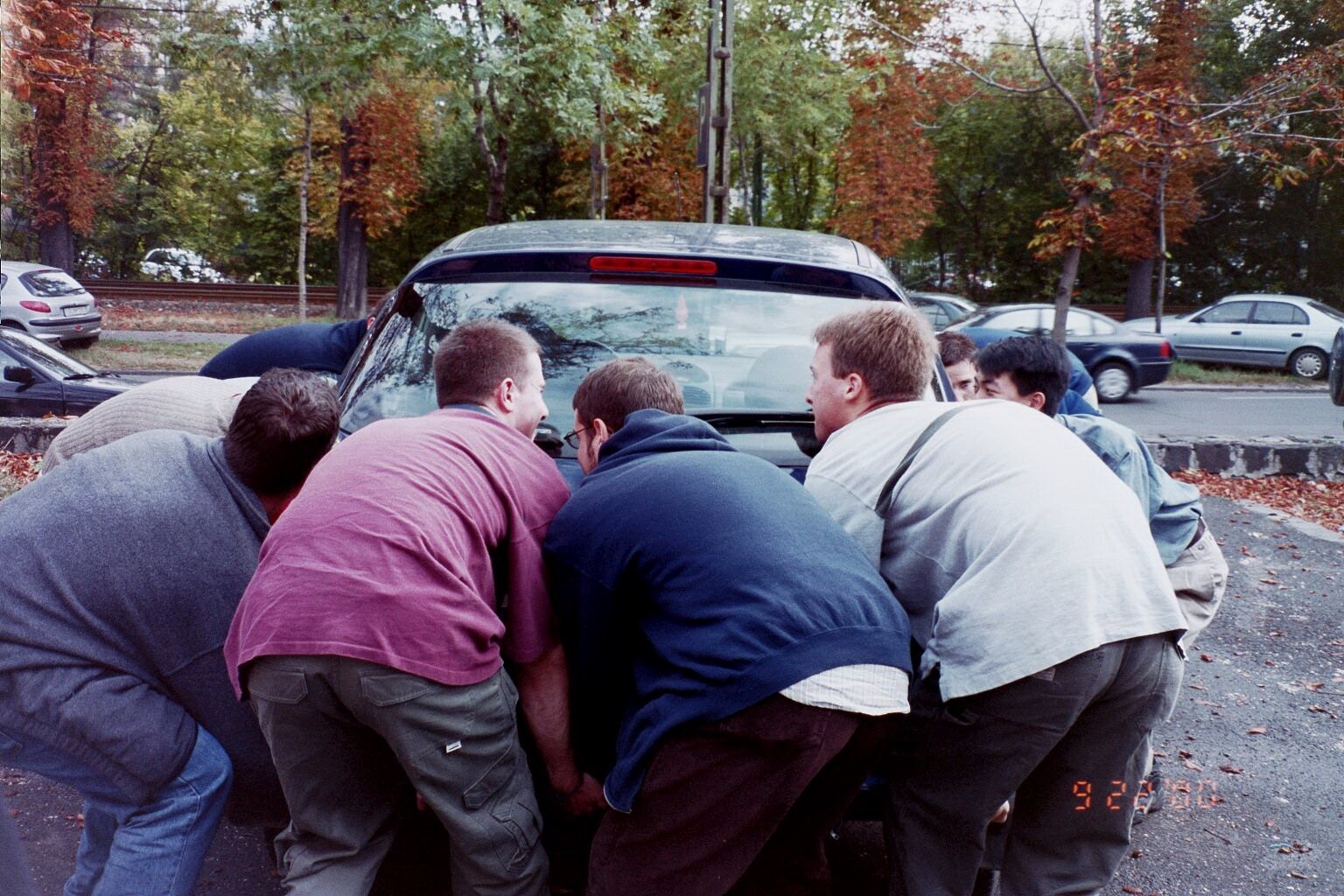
[555,769,584,800]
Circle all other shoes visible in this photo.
[1137,761,1162,813]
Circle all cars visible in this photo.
[139,247,226,282]
[0,323,200,417]
[935,300,1179,406]
[907,290,981,331]
[0,259,104,351]
[325,219,1013,895]
[1121,291,1344,381]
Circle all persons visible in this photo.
[803,301,1229,896]
[0,319,606,896]
[543,356,911,896]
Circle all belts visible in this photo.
[1186,518,1205,551]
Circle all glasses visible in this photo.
[564,428,586,450]
[336,427,353,445]
